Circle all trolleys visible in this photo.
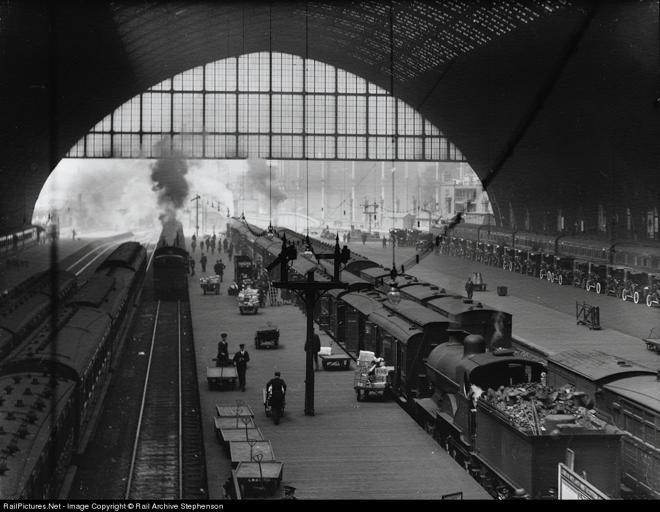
[210,400,257,418]
[210,345,244,366]
[203,358,240,391]
[218,417,267,442]
[210,413,259,430]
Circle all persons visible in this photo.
[304,327,321,371]
[233,344,250,392]
[188,230,234,282]
[72,228,77,240]
[266,371,286,425]
[465,277,473,298]
[361,231,367,245]
[383,236,387,248]
[217,333,229,361]
[343,231,351,244]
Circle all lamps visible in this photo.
[387,0,401,306]
[266,2,275,241]
[300,3,314,261]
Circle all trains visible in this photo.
[151,217,197,300]
[0,242,146,500]
[0,268,77,359]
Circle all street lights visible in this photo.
[190,193,201,230]
